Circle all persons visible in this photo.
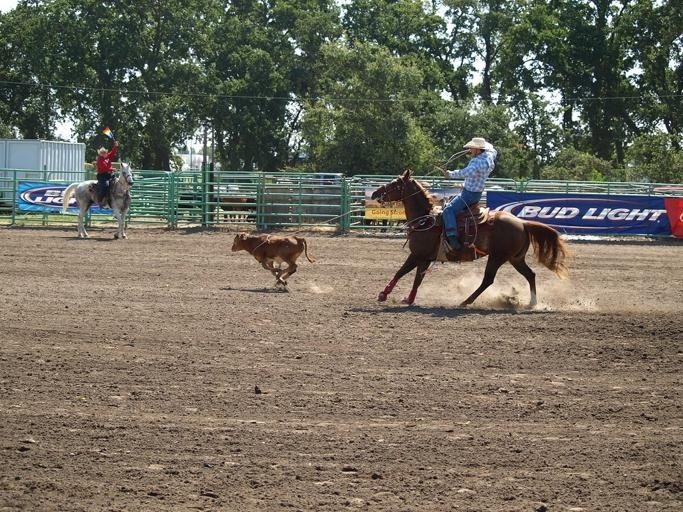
[441,137,498,250]
[96,140,119,206]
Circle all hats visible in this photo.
[97,147,108,154]
[463,137,494,150]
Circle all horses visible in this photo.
[370,167,574,311]
[62,161,135,240]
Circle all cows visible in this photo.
[230,232,316,285]
[170,190,257,229]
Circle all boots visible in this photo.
[446,237,471,260]
